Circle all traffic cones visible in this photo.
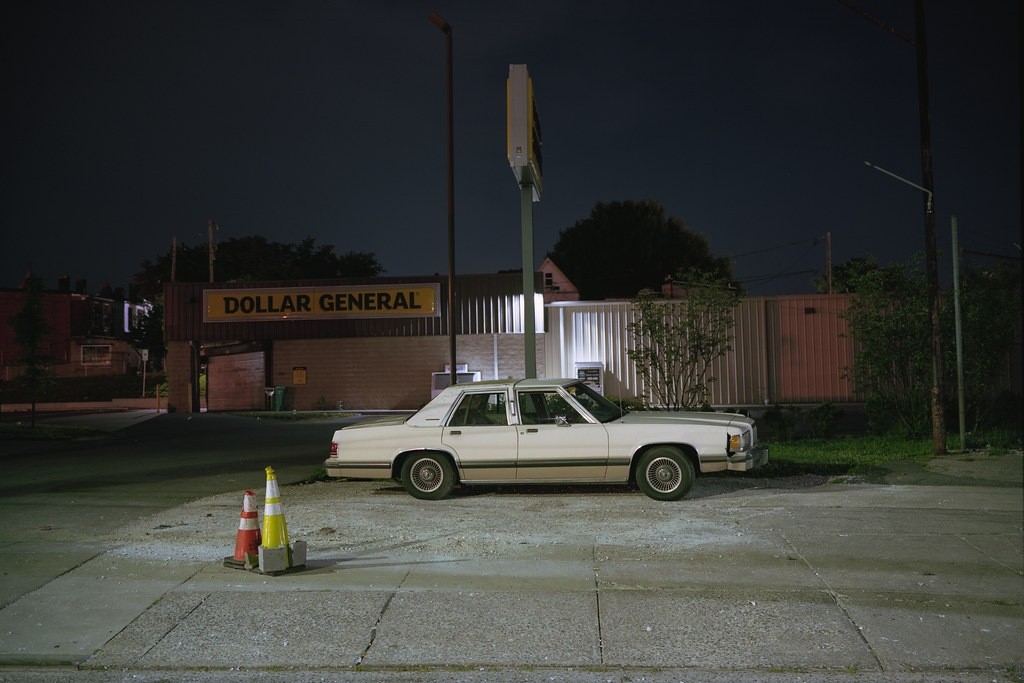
[223,488,262,566]
[259,465,293,572]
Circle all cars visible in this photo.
[324,378,770,501]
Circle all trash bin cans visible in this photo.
[272,387,296,411]
[575,362,605,398]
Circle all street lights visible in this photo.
[427,9,455,385]
